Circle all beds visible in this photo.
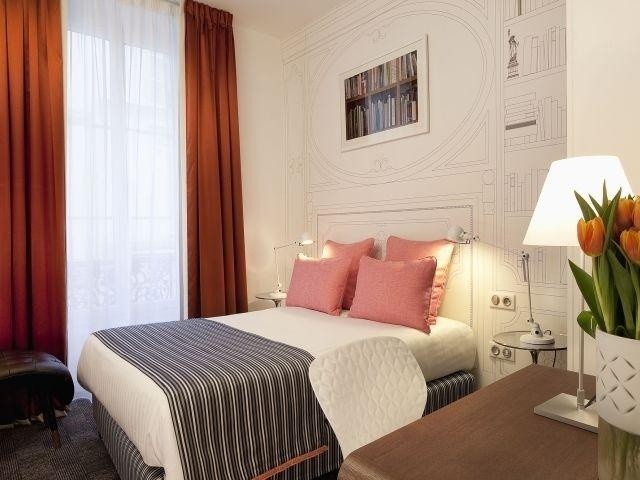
[73,305,488,480]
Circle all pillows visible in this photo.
[383,234,454,324]
[347,255,437,335]
[284,253,354,315]
[319,238,377,309]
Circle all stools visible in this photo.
[1,344,74,448]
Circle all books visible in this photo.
[339,52,420,143]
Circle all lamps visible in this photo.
[447,225,555,344]
[272,233,316,298]
[523,156,637,435]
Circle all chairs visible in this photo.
[309,338,430,459]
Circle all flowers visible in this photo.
[568,186,639,348]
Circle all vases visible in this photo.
[597,326,640,480]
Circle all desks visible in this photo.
[255,292,287,306]
[493,328,573,366]
[336,368,596,480]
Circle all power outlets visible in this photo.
[489,291,515,310]
[490,344,516,363]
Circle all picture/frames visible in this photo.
[338,35,431,153]
